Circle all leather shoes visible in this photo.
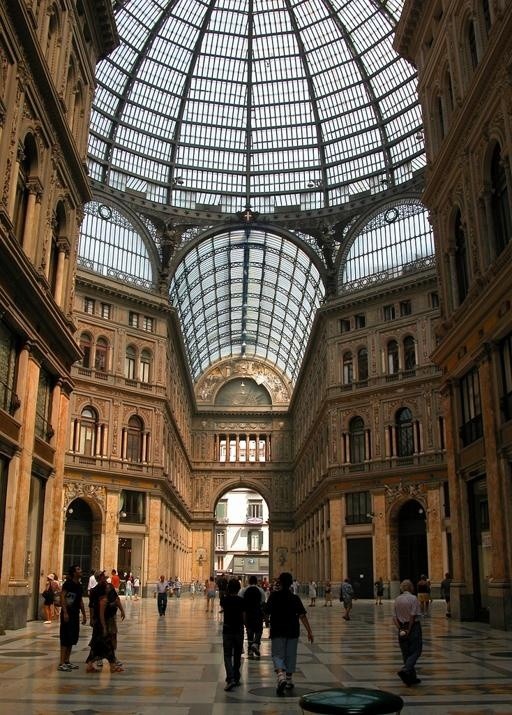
[413,678,421,684]
[397,671,413,687]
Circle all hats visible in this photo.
[47,574,55,580]
[95,570,106,579]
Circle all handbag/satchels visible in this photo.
[42,590,50,599]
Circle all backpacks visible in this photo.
[243,586,261,613]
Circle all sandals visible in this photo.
[87,667,101,672]
[110,666,124,673]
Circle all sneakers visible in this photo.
[224,681,236,691]
[251,643,260,656]
[65,663,79,669]
[97,659,103,666]
[44,620,51,624]
[277,679,287,693]
[115,660,123,666]
[56,664,71,672]
[248,652,254,657]
[286,680,294,688]
[342,616,350,620]
